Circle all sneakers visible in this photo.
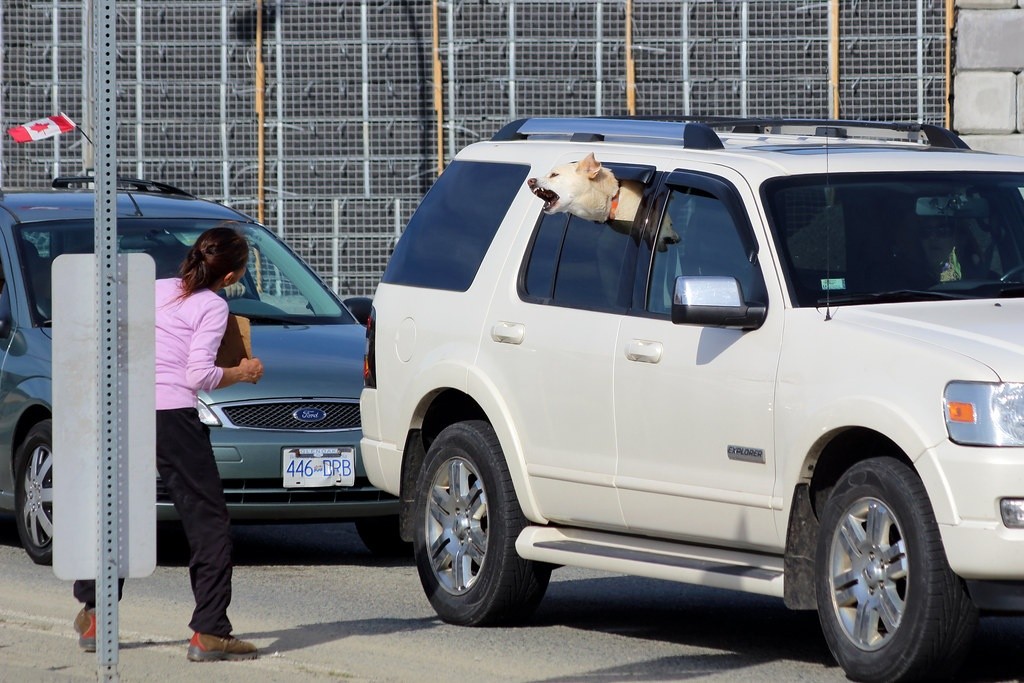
[186,631,258,661]
[74,607,96,651]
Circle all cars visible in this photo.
[0,168,414,567]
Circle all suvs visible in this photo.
[358,113,1024,683]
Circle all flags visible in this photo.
[7,112,76,143]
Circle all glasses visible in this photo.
[922,222,955,239]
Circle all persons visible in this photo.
[915,215,1002,296]
[73,224,262,665]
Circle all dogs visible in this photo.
[528,151,643,223]
[644,211,681,252]
